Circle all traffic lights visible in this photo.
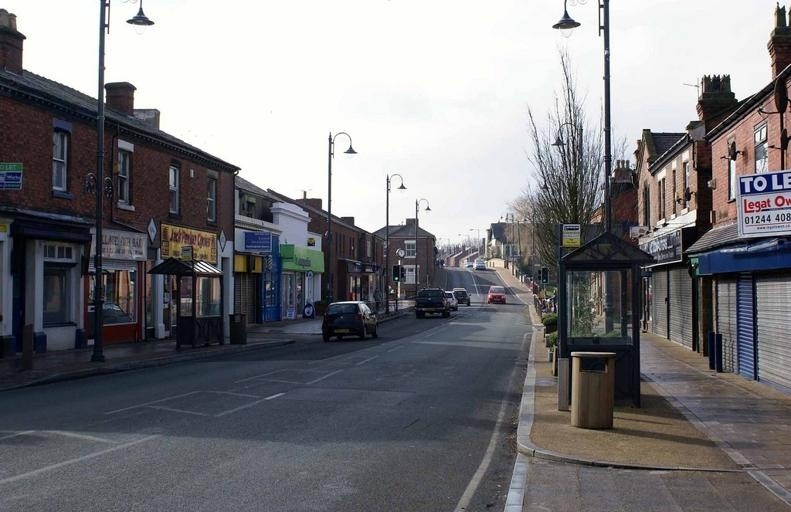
[393,266,399,280]
[401,266,407,281]
[541,268,547,283]
[537,269,542,282]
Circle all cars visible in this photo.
[486,285,507,304]
[387,284,396,300]
[321,301,381,341]
[414,285,471,320]
[465,260,488,271]
[171,298,193,323]
[87,303,131,327]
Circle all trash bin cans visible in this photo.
[228,314,247,344]
[570,351,617,429]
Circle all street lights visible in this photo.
[385,171,408,314]
[414,194,433,299]
[437,227,482,258]
[324,130,358,309]
[536,0,620,233]
[86,0,158,361]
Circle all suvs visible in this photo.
[436,259,445,266]
